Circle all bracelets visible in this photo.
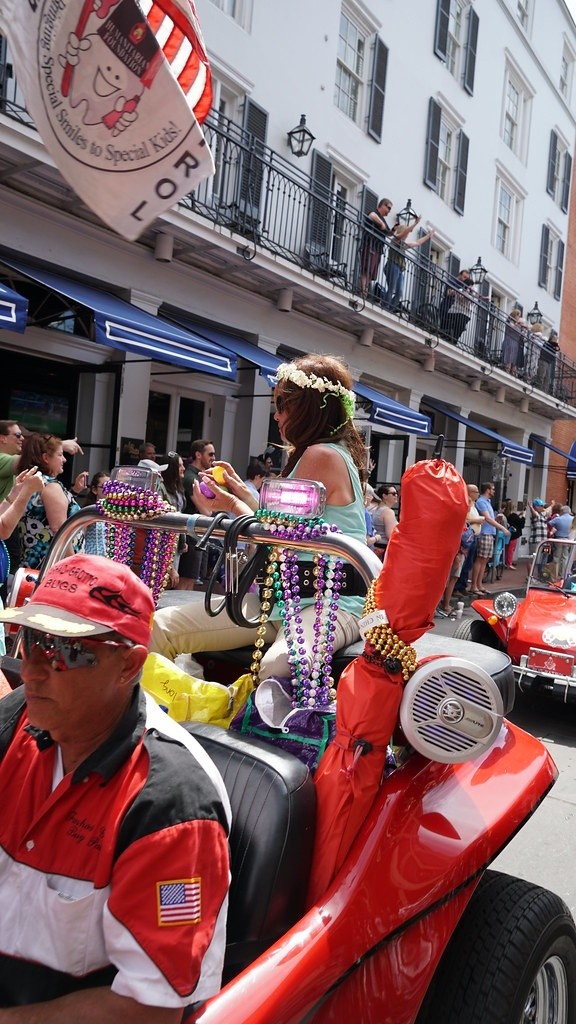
[5,496,13,504]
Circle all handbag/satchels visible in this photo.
[461,528,475,547]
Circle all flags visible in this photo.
[0,0,216,242]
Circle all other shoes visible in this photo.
[434,564,518,618]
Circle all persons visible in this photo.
[360,198,393,299]
[385,214,436,312]
[0,420,110,655]
[442,482,576,616]
[147,353,367,683]
[0,554,232,1024]
[363,481,399,559]
[139,440,277,591]
[437,270,490,347]
[502,308,560,392]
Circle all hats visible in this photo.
[533,500,546,507]
[1,553,155,649]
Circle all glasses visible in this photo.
[202,452,216,457]
[276,396,286,414]
[168,450,177,462]
[4,432,23,438]
[18,625,127,672]
[387,492,398,496]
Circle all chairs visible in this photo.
[169,720,309,995]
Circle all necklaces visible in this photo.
[97,479,179,612]
[252,509,417,714]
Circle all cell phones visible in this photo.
[84,475,88,486]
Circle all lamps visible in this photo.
[154,233,174,263]
[529,301,542,326]
[359,327,375,346]
[423,350,436,373]
[288,114,315,157]
[520,397,529,414]
[493,387,507,404]
[471,376,482,393]
[469,255,486,286]
[398,198,417,232]
[278,288,293,312]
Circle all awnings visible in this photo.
[421,400,535,465]
[350,379,431,436]
[0,252,284,398]
[530,436,576,479]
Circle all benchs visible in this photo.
[213,200,262,239]
[191,632,514,717]
[304,242,350,289]
[375,283,409,316]
[480,340,504,361]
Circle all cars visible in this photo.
[0,467,576,1024]
[452,536,576,696]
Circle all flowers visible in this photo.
[275,362,358,402]
